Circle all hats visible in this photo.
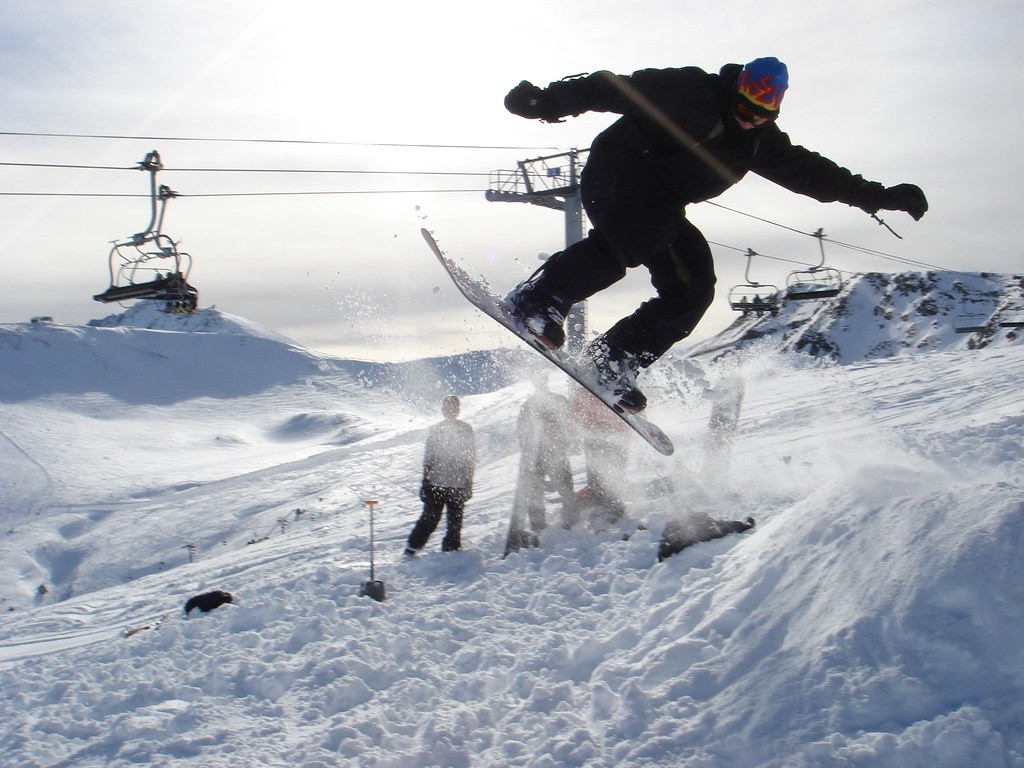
[738,57,789,118]
[443,395,459,409]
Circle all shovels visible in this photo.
[503,433,540,558]
[358,500,386,601]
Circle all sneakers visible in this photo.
[586,334,647,414]
[510,280,569,350]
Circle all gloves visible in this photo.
[463,481,472,501]
[876,182,929,221]
[503,79,561,122]
[419,480,431,503]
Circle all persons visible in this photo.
[503,57,928,410]
[753,294,763,316]
[402,395,475,556]
[516,368,631,530]
[157,272,198,313]
[741,296,748,315]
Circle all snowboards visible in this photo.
[419,227,676,458]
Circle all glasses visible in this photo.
[735,100,771,127]
